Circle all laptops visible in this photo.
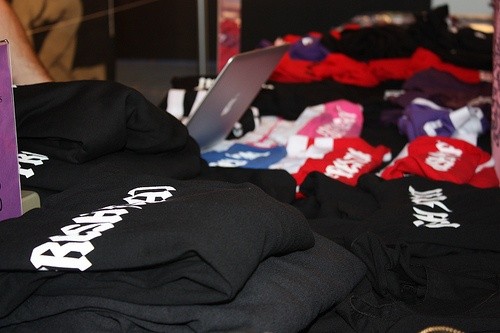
[185,43,291,151]
